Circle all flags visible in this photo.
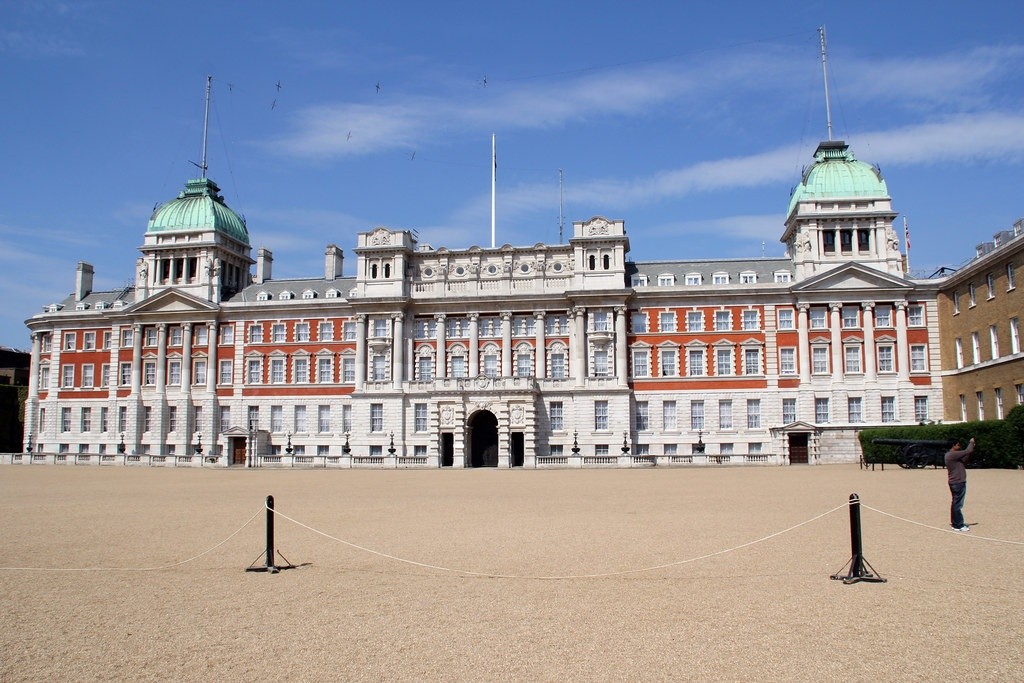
[905,225,910,249]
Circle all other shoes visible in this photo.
[952,526,970,532]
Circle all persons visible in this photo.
[944,438,975,532]
